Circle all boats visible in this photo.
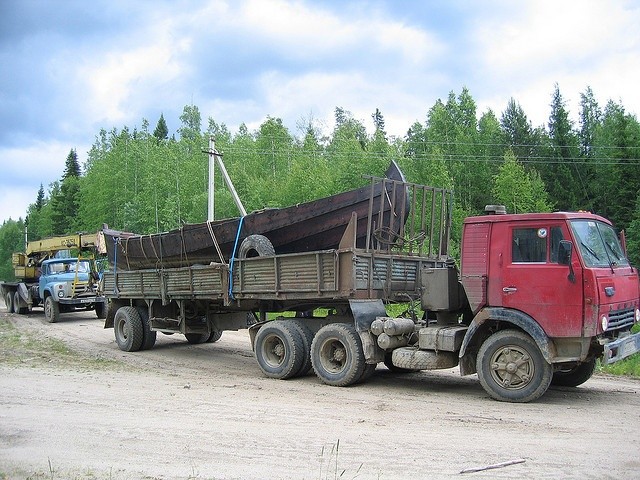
[103,160,410,266]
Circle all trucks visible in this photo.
[1,222,108,323]
[102,159,640,402]
